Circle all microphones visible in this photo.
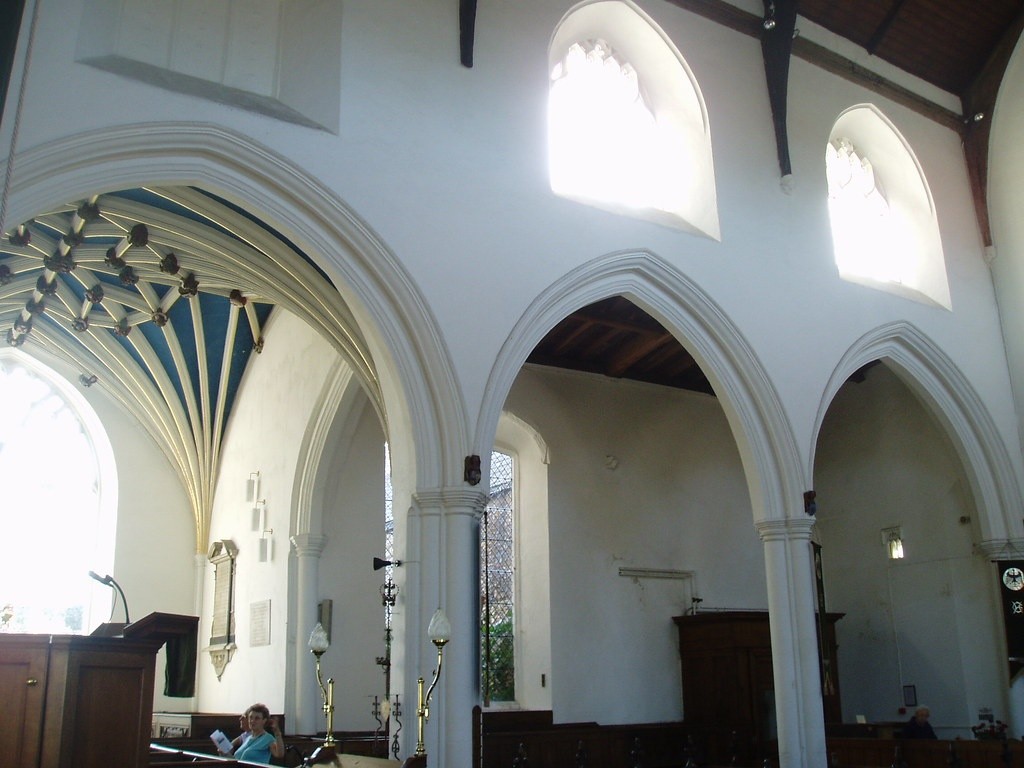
[88,571,114,587]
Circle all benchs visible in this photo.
[826,736,1024,768]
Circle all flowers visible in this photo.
[972,720,1008,741]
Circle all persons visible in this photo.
[218,704,285,764]
[1009,668,1024,741]
[911,706,938,739]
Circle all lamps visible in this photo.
[373,557,400,570]
[404,606,451,768]
[880,526,904,559]
[304,622,343,768]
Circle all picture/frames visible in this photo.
[903,685,917,707]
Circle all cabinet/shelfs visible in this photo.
[671,611,847,768]
